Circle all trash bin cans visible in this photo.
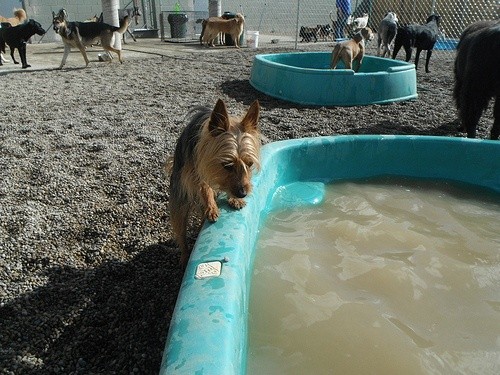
[220,11,243,45]
[168,13,189,38]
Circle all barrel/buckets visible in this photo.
[246,30,259,48]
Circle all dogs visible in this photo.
[330,27,375,73]
[347,28,364,40]
[195,16,229,45]
[317,23,333,41]
[98,5,143,44]
[377,11,399,58]
[0,19,46,68]
[346,15,354,25]
[201,13,245,49]
[392,14,442,73]
[167,97,261,271]
[299,32,318,43]
[329,10,359,40]
[353,13,369,29]
[452,19,500,142]
[0,8,27,29]
[301,26,320,40]
[52,8,128,69]
[82,14,97,48]
